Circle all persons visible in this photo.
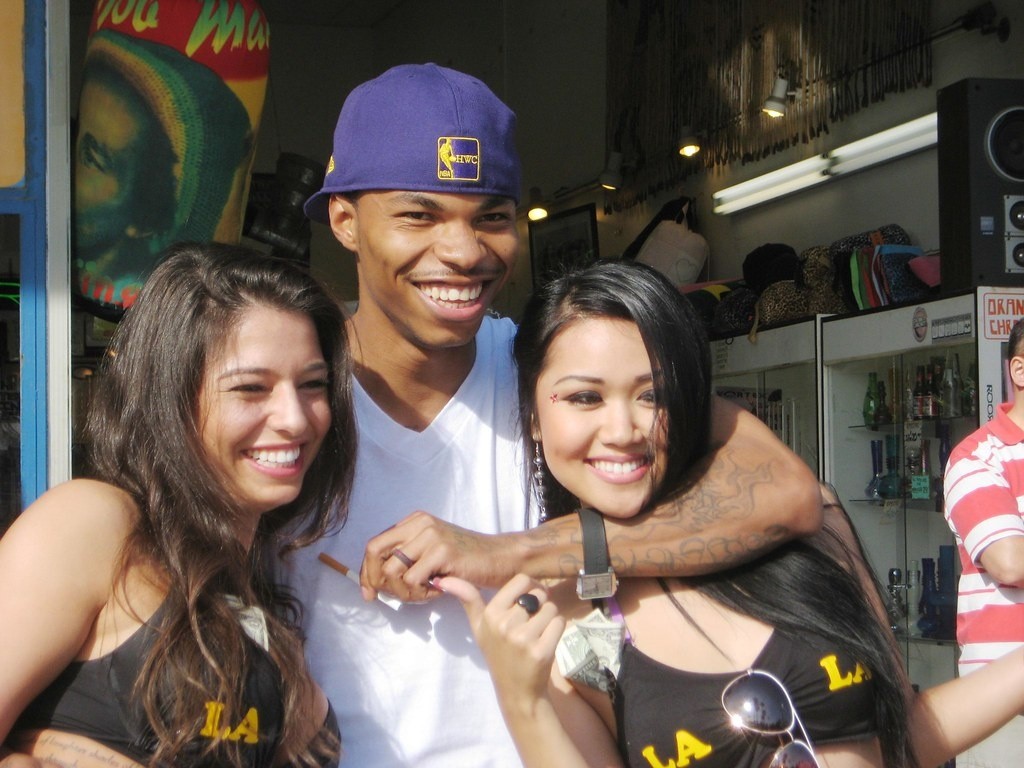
[433,259,1024,767]
[68,66,177,275]
[943,316,1024,768]
[0,238,359,768]
[253,63,822,767]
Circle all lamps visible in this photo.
[760,77,802,118]
[677,126,709,157]
[599,151,637,192]
[240,151,325,258]
[710,152,837,216]
[829,111,938,179]
[526,186,556,221]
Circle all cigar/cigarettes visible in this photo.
[317,553,402,611]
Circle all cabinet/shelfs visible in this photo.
[820,285,1024,693]
[708,313,836,483]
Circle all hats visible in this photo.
[304,63,522,224]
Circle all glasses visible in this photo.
[722,667,821,768]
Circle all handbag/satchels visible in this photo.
[713,224,941,343]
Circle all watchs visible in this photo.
[576,508,619,600]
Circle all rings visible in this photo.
[394,550,414,568]
[518,594,539,613]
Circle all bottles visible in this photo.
[863,353,977,423]
[887,544,956,638]
[866,433,951,499]
